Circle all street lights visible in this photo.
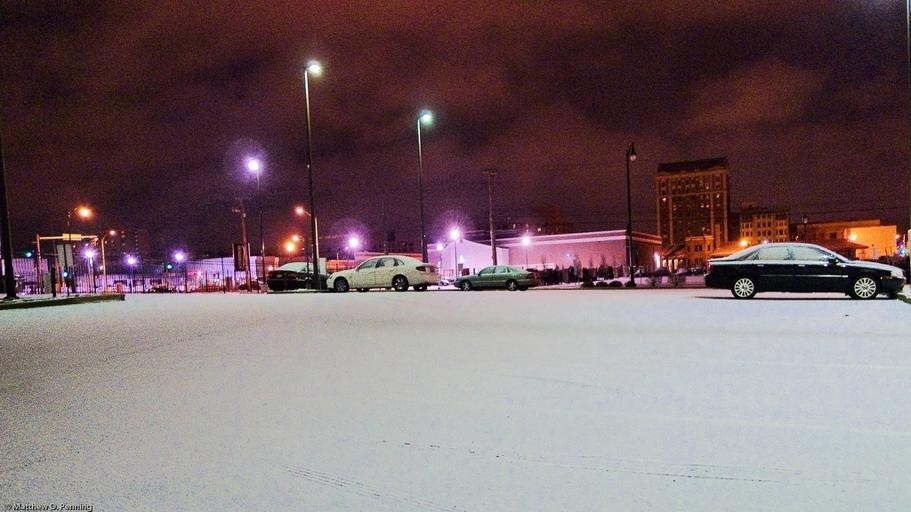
[250,160,267,291]
[524,236,528,268]
[68,206,188,293]
[336,238,357,272]
[626,142,637,286]
[302,59,323,290]
[438,230,458,279]
[416,109,433,290]
[801,213,808,243]
[295,206,311,219]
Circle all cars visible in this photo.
[267,255,541,292]
[630,266,706,277]
[703,242,906,300]
